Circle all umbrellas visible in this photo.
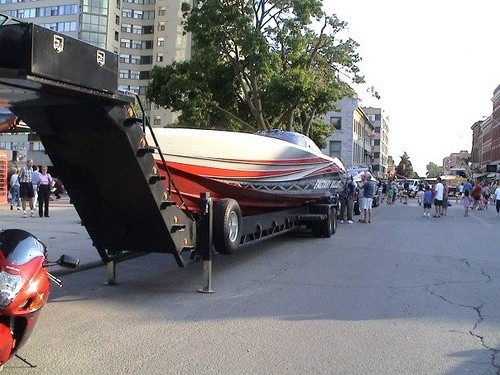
[353,171,376,182]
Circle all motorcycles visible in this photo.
[0,227,80,374]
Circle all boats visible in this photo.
[141,126,350,213]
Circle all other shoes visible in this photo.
[23,212,27,218]
[17,207,20,210]
[30,212,36,218]
[10,204,14,210]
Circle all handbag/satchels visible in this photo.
[14,185,21,195]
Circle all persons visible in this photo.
[338,171,500,225]
[9,159,64,218]
